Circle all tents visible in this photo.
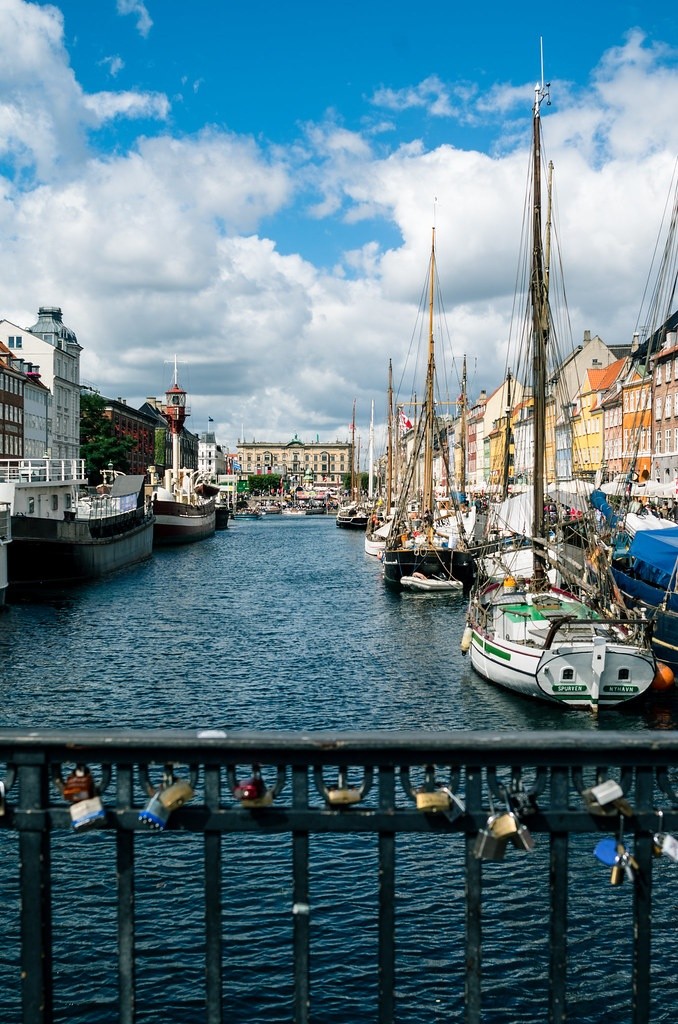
[435,478,678,504]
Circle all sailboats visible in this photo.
[459,83,675,713]
[335,398,383,527]
[365,161,678,667]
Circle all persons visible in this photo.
[219,486,678,529]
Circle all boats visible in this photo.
[95,354,216,537]
[234,508,262,521]
[259,507,281,514]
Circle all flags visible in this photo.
[349,424,357,430]
[399,405,412,429]
[208,415,214,422]
